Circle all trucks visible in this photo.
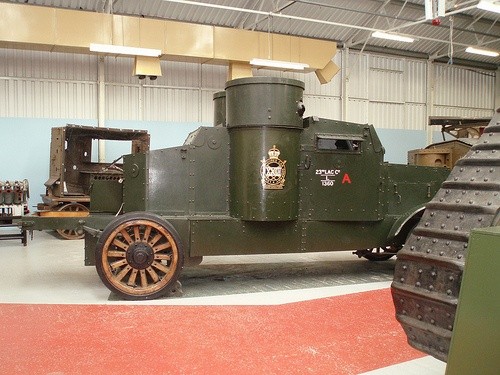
[18,76,454,300]
[33,124,152,240]
